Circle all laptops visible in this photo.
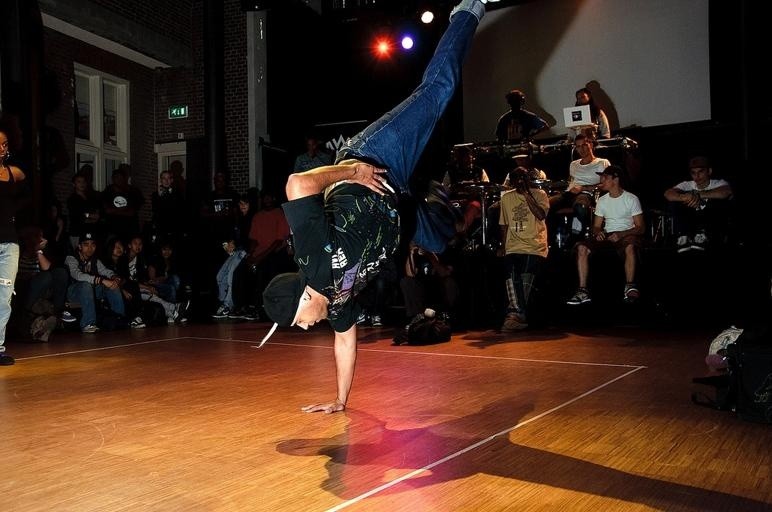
[563,104,599,128]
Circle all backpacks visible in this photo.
[691,331,772,425]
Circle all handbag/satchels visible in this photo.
[405,308,451,346]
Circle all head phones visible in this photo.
[511,90,526,105]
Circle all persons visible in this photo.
[264,0,498,414]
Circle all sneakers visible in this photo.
[166,310,187,323]
[691,232,707,250]
[81,325,101,333]
[173,300,190,319]
[0,352,15,365]
[504,314,529,330]
[128,317,146,328]
[624,283,640,304]
[58,310,77,323]
[211,305,261,321]
[677,234,692,253]
[566,287,592,304]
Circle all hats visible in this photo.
[261,269,305,329]
[78,232,94,242]
[688,157,709,168]
[596,165,622,178]
